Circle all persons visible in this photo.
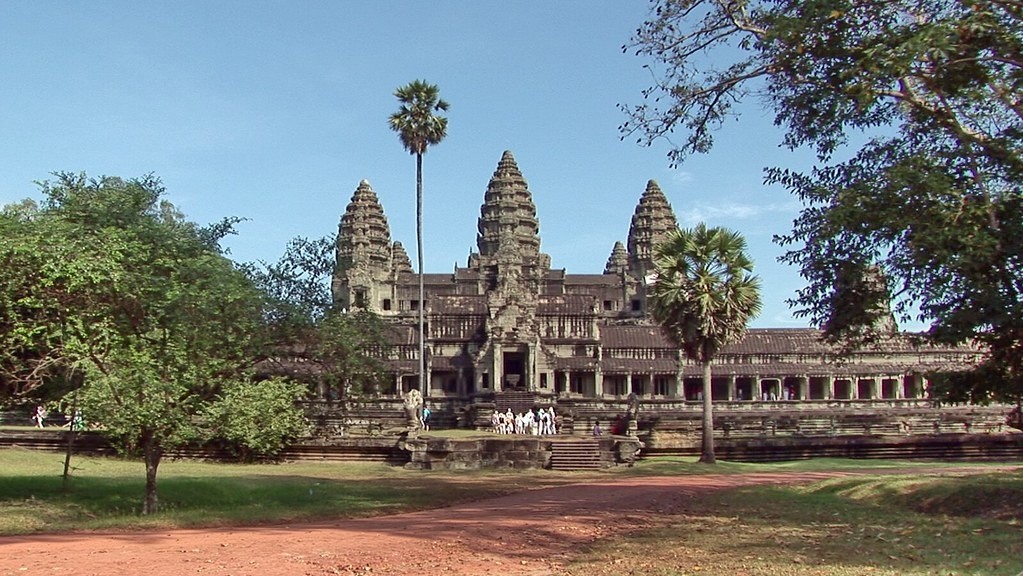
[423,404,432,430]
[491,406,556,435]
[36,405,47,427]
[762,390,769,401]
[737,389,744,400]
[594,421,602,436]
[790,384,797,399]
[770,384,776,401]
